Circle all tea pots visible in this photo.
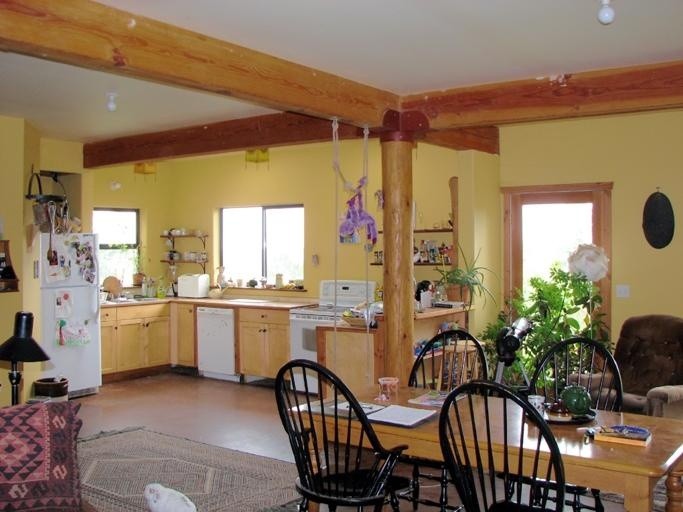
[560,383,591,418]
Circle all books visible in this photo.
[406,389,468,409]
[305,395,438,429]
[592,427,652,448]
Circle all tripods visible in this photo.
[488,352,530,398]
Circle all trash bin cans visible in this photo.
[34,376,69,403]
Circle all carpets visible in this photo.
[75,425,311,511]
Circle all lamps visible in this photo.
[1,311,52,406]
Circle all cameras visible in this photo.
[496,317,533,355]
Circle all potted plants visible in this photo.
[106,225,152,286]
[429,241,502,317]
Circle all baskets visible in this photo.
[343,317,367,326]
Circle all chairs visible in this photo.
[564,313,683,419]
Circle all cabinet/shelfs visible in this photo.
[100,307,118,385]
[118,304,170,381]
[233,307,290,388]
[159,233,209,274]
[370,227,453,267]
[174,303,198,377]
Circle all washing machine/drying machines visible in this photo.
[289,281,374,393]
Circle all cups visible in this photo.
[528,395,544,419]
[376,375,399,404]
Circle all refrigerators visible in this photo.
[38,233,102,399]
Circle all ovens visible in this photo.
[288,314,342,395]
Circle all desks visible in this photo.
[283,384,683,512]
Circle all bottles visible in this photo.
[141,274,168,298]
[372,238,453,264]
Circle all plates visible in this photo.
[366,404,436,432]
